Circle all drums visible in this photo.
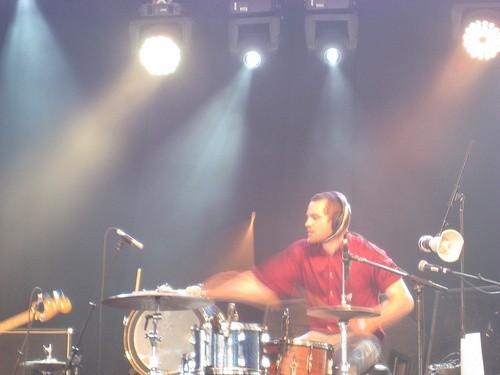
[261,340,335,375]
[191,319,268,375]
[123,291,226,375]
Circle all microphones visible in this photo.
[116,228,145,249]
[418,261,450,275]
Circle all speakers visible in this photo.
[0,329,73,375]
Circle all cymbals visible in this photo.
[305,304,381,320]
[101,289,213,310]
[18,357,68,375]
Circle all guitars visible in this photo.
[0,289,72,334]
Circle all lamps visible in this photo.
[224,0,285,73]
[303,0,361,69]
[127,0,197,80]
[449,0,500,66]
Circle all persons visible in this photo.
[203,190,414,375]
